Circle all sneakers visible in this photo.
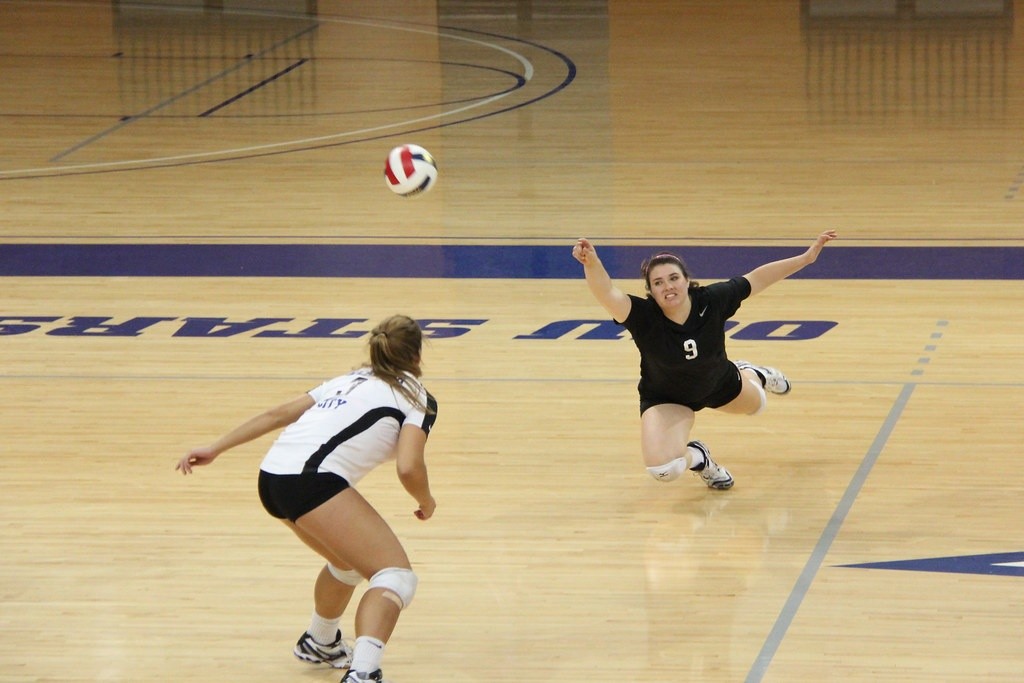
[340,667,383,683]
[734,360,792,395]
[686,440,734,490]
[294,628,354,668]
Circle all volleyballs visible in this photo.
[384,144,437,198]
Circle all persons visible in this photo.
[571,229,838,490]
[176,314,438,683]
